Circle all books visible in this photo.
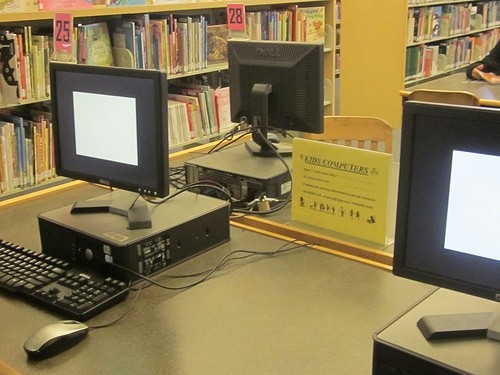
[167,82,236,147]
[475,69,500,85]
[405,0,500,88]
[0,6,326,105]
[0,101,58,194]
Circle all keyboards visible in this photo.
[0,239,129,323]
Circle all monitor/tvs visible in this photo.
[49,62,170,229]
[392,100,500,341]
[227,37,324,157]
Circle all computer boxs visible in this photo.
[371,286,500,375]
[36,186,231,285]
[184,140,293,213]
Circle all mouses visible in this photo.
[23,320,90,360]
[267,132,279,143]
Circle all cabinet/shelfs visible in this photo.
[0,0,344,202]
[339,0,500,126]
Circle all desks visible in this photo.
[398,71,500,110]
[0,134,438,375]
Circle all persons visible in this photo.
[467,39,500,80]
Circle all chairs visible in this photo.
[305,91,479,158]
